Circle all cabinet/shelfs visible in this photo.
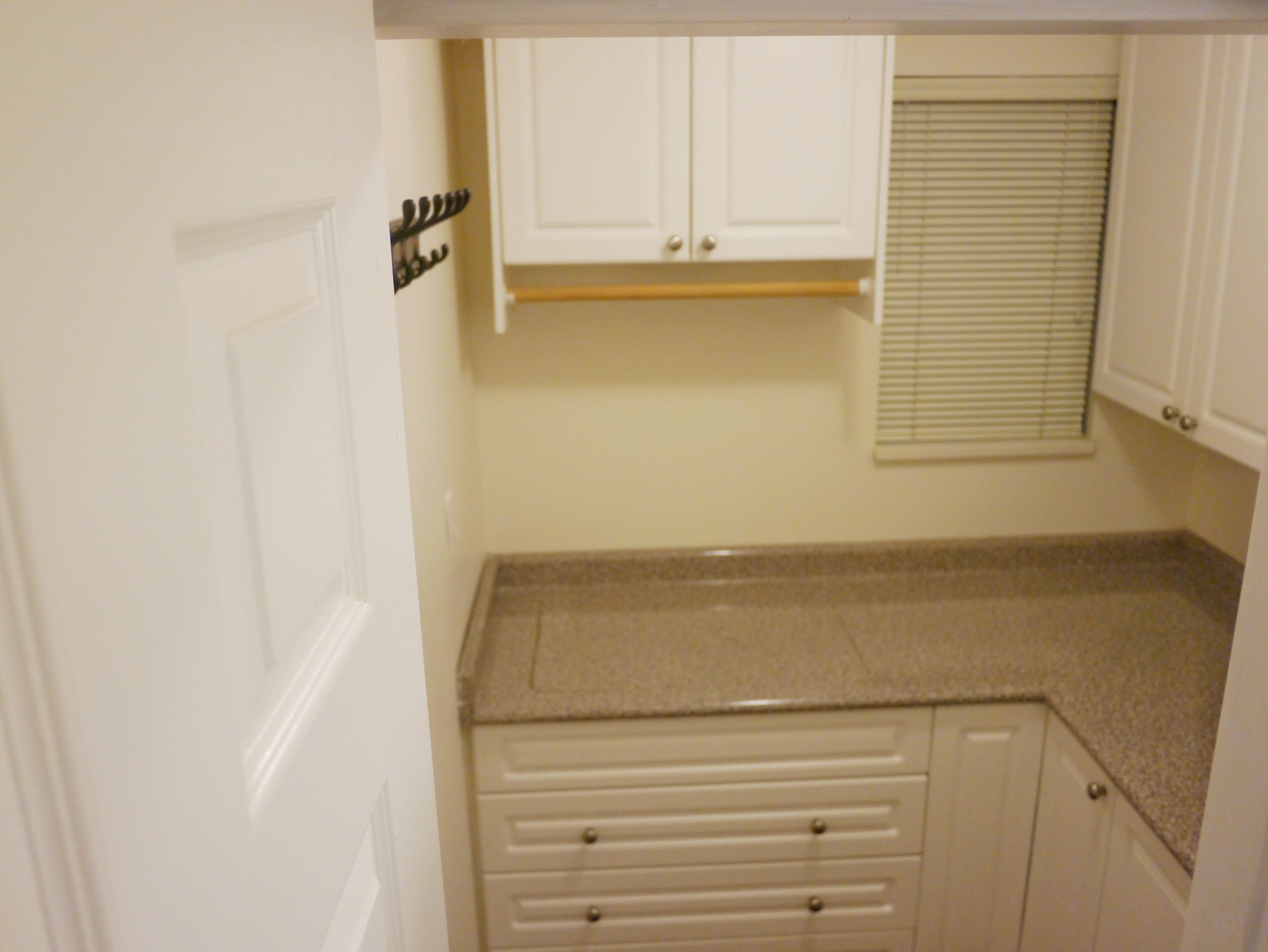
[1091,33,1266,469]
[469,701,1194,952]
[482,35,895,334]
